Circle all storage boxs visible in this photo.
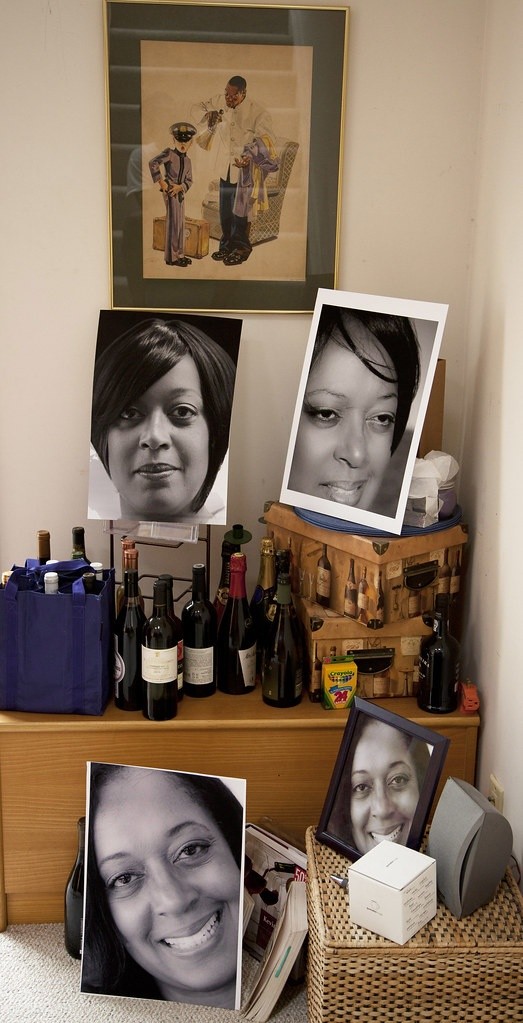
[265,503,468,700]
[347,840,436,945]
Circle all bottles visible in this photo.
[140,580,177,720]
[116,536,144,616]
[438,480,457,518]
[313,643,420,702]
[378,547,461,624]
[216,554,258,695]
[113,569,145,712]
[418,593,463,713]
[144,574,184,705]
[181,564,216,696]
[249,537,278,634]
[214,525,248,625]
[1,527,102,597]
[288,537,370,618]
[258,548,305,708]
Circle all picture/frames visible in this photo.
[102,0,350,314]
[316,696,450,860]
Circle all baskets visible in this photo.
[306,824,523,1023]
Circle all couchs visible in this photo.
[201,131,299,244]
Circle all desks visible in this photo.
[1,675,490,935]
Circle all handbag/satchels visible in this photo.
[2,559,117,716]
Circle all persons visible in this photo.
[287,303,421,512]
[81,762,243,1010]
[91,319,236,521]
[324,709,430,868]
